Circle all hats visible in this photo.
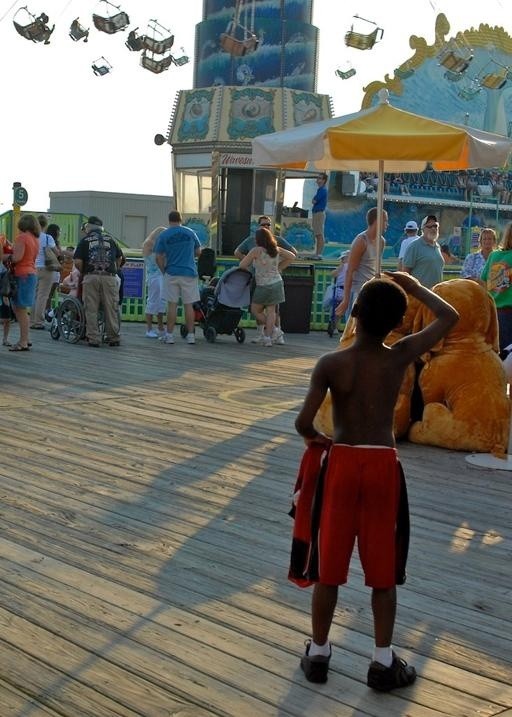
[405,221,419,230]
[422,215,438,225]
[88,216,102,226]
[337,250,351,261]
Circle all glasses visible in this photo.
[260,223,270,226]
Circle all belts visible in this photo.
[89,271,115,276]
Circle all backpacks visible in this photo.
[198,248,215,275]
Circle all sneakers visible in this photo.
[367,650,417,690]
[300,639,332,684]
[88,328,285,348]
[30,308,52,329]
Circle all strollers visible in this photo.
[178,265,249,344]
[44,276,122,342]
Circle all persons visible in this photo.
[453,167,511,205]
[138,225,169,339]
[205,277,221,290]
[457,226,498,283]
[440,248,463,267]
[152,208,203,345]
[237,225,296,348]
[294,270,460,693]
[396,218,422,271]
[0,206,129,352]
[402,212,447,289]
[308,172,329,261]
[329,249,355,336]
[358,170,414,197]
[35,12,55,45]
[332,205,387,318]
[479,221,512,361]
[233,213,298,345]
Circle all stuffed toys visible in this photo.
[408,273,512,459]
[313,271,422,439]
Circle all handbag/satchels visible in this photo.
[1,271,18,297]
[43,246,62,272]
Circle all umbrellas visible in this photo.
[252,87,512,279]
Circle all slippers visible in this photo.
[3,341,33,353]
